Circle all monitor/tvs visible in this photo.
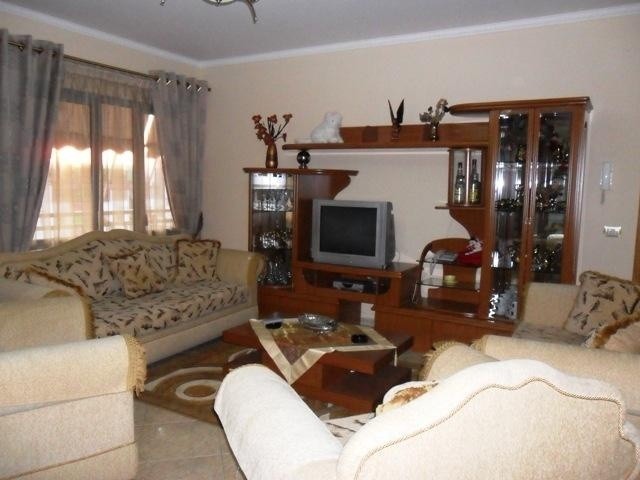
[311,199,397,269]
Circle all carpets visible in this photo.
[133,339,428,427]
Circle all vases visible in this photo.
[265,143,279,168]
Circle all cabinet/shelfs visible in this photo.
[257,257,523,350]
[244,166,359,289]
[413,238,481,319]
[445,96,593,331]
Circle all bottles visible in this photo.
[470,159,483,206]
[454,162,465,204]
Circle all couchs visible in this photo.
[476,270,640,432]
[1,225,267,364]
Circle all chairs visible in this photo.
[0,294,143,479]
[211,338,640,480]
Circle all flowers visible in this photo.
[252,112,293,143]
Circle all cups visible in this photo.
[444,275,456,286]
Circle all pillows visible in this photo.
[563,271,639,338]
[104,241,166,299]
[578,312,640,357]
[175,238,220,286]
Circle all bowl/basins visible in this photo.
[298,312,336,334]
